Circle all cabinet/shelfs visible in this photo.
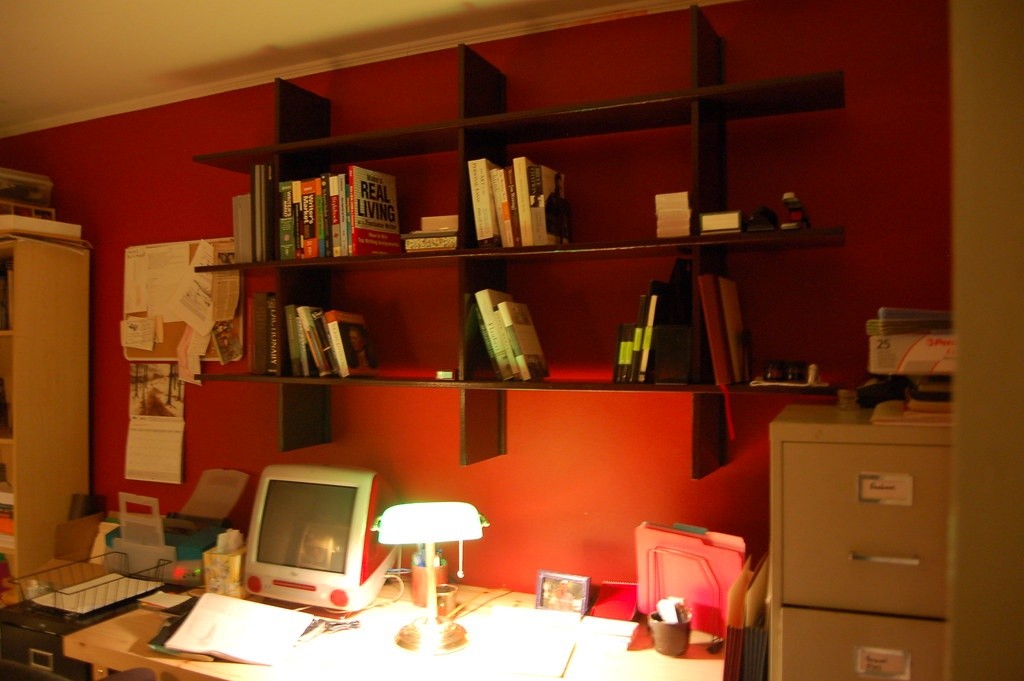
[0,575,171,681]
[0,239,89,608]
[769,401,953,681]
[193,4,843,479]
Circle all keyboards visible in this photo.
[298,620,326,640]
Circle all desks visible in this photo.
[64,581,726,681]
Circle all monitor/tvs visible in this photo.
[244,463,401,613]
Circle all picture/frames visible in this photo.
[536,570,591,613]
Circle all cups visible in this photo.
[410,561,449,607]
[648,611,693,656]
[435,584,459,616]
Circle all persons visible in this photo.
[549,585,572,610]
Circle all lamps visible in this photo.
[373,501,490,657]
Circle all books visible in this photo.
[260,153,750,386]
[138,590,328,667]
[870,373,957,428]
